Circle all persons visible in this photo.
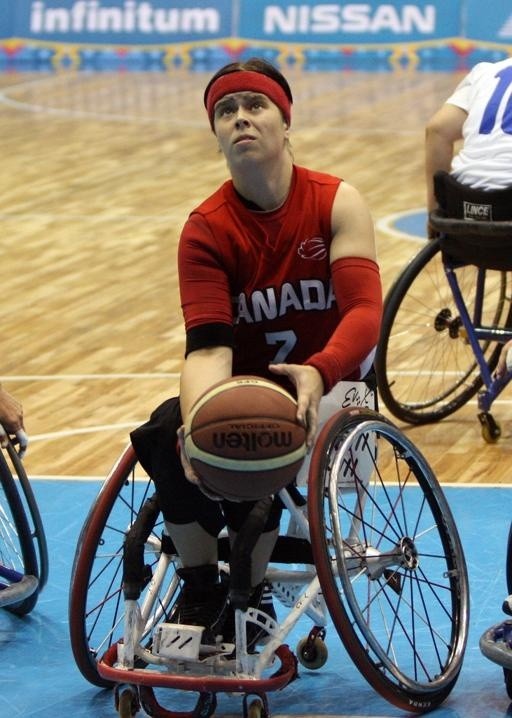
[1,383,29,460]
[422,55,512,244]
[494,337,512,618]
[126,54,391,666]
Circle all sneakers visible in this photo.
[173,566,227,643]
[209,581,278,659]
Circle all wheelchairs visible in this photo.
[372,170,511,446]
[0,416,51,616]
[67,366,472,718]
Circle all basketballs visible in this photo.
[180,374,311,506]
